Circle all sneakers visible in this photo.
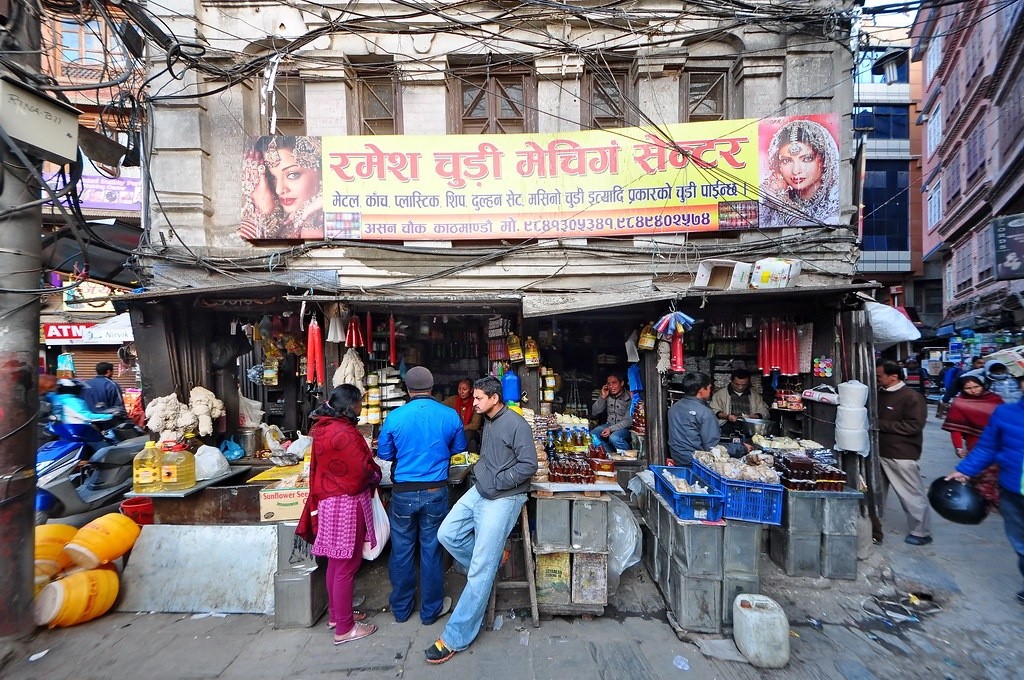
[424,638,456,663]
[498,550,509,569]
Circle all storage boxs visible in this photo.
[258,481,310,521]
[650,455,786,525]
[750,256,803,290]
[692,259,752,291]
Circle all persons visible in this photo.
[711,366,770,451]
[890,351,932,398]
[423,375,538,664]
[590,372,633,457]
[440,372,483,460]
[941,351,1024,513]
[864,356,935,546]
[376,365,466,630]
[758,117,837,229]
[242,134,326,244]
[662,372,753,473]
[39,361,127,452]
[945,368,1023,607]
[296,384,382,647]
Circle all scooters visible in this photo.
[34,377,150,529]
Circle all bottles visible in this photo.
[636,311,695,351]
[257,425,292,457]
[358,371,382,425]
[230,318,307,386]
[132,432,205,493]
[303,437,313,482]
[369,319,408,359]
[538,365,563,415]
[489,331,524,417]
[535,426,608,485]
[525,335,540,368]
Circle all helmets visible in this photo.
[905,358,918,368]
[927,476,988,526]
[55,377,92,394]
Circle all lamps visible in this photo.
[871,39,908,84]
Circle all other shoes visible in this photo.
[422,597,452,625]
[904,534,932,545]
[1016,591,1024,605]
[935,414,939,417]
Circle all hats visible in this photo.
[405,366,434,390]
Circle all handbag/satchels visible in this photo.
[361,486,390,561]
[238,388,266,427]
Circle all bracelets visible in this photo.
[256,203,284,240]
[777,202,803,224]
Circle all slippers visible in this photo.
[333,620,379,645]
[327,610,367,629]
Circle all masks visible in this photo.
[991,373,1006,380]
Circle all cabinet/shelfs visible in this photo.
[704,337,758,398]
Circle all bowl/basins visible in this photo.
[737,418,777,437]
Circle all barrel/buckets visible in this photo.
[35,497,154,630]
[238,426,263,457]
[733,593,790,668]
